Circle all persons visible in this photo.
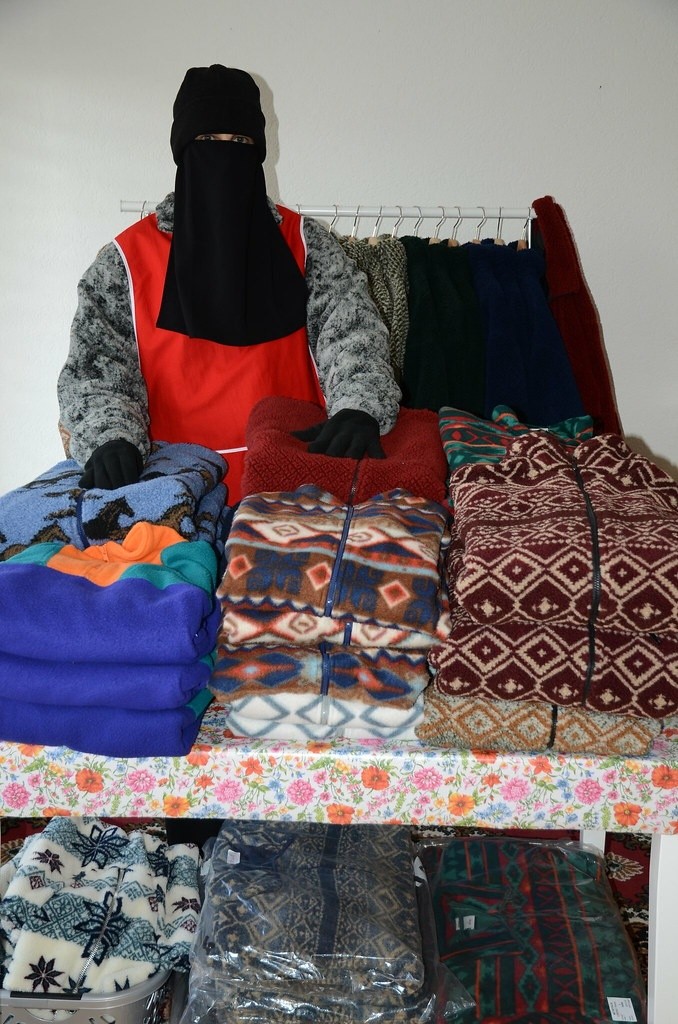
[58,64,402,491]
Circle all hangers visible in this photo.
[295,203,531,250]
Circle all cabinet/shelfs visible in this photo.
[0,739,678,1024]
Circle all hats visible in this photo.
[168,63,267,164]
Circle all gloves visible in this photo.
[77,441,144,489]
[290,409,386,462]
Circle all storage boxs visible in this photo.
[0,971,169,1024]
